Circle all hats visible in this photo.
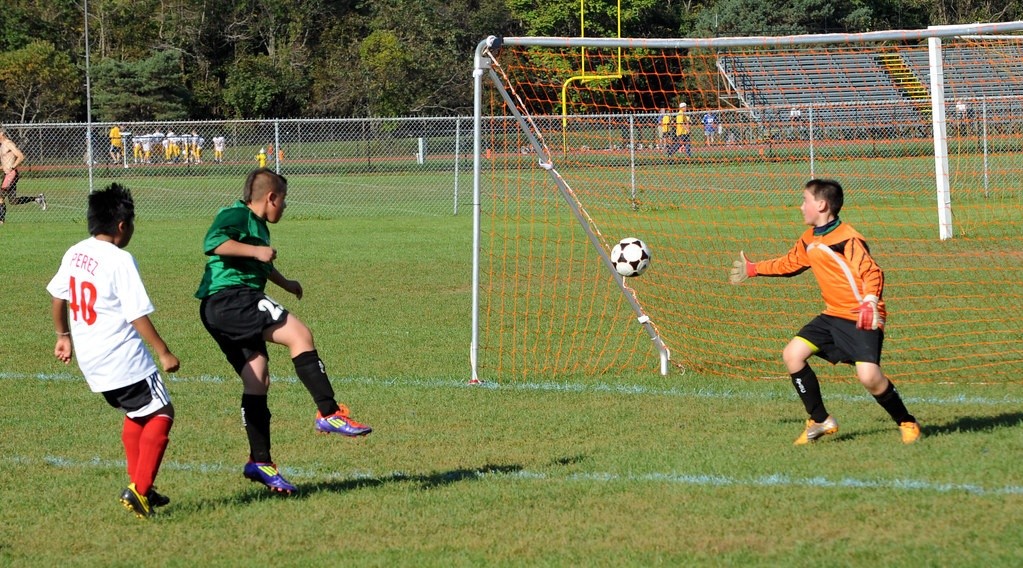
[679,102,686,108]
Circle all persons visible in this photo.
[110,124,124,164]
[162,127,180,163]
[46,181,181,519]
[957,101,966,120]
[267,142,285,162]
[0,126,46,222]
[790,108,804,130]
[132,130,154,164]
[213,134,225,163]
[181,129,205,164]
[731,179,921,445]
[658,102,692,157]
[193,167,371,492]
[152,127,166,155]
[726,129,735,148]
[702,109,718,146]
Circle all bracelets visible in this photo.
[56,331,69,336]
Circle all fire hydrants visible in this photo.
[256,148,267,168]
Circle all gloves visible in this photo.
[729,250,757,284]
[848,294,880,330]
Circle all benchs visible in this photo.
[717,46,1023,136]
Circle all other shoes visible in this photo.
[113,160,123,165]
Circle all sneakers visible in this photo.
[35,193,47,211]
[314,403,372,438]
[119,483,156,521]
[792,413,839,445]
[243,454,297,494]
[898,421,921,446]
[144,489,171,508]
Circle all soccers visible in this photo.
[610,236,651,277]
